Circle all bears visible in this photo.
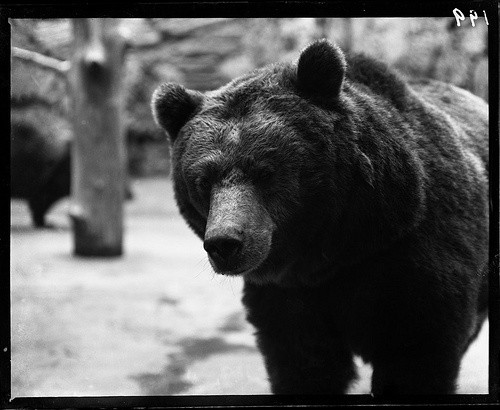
[150,38,489,395]
